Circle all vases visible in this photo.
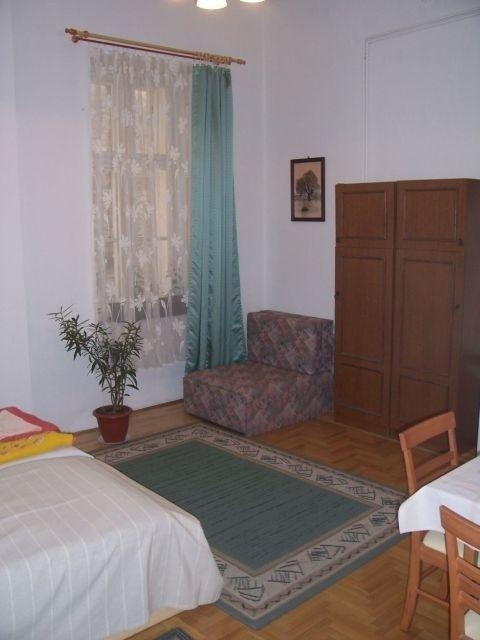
[47,304,143,445]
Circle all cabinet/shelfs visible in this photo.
[334,179,479,455]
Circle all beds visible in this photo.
[0,408,224,639]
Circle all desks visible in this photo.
[398,455,480,571]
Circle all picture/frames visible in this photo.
[291,158,325,222]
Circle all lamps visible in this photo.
[197,0,229,10]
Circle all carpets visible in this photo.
[86,422,412,631]
[154,627,195,639]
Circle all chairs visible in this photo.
[439,506,480,640]
[398,411,480,633]
[184,311,334,435]
